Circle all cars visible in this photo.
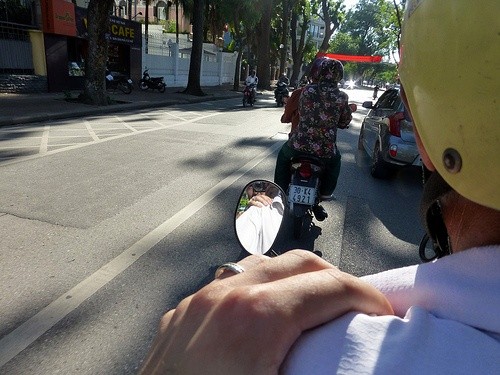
[359,88,424,180]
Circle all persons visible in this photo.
[373,85,379,97]
[242,70,258,102]
[137,249,394,375]
[274,58,352,200]
[294,78,299,88]
[274,73,290,102]
[278,0,500,375]
[236,181,274,219]
[301,76,309,88]
[236,183,285,255]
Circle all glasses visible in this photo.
[249,182,269,192]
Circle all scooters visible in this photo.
[285,103,358,240]
[105,68,135,95]
[274,78,289,107]
[138,70,167,94]
[242,78,258,108]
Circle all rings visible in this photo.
[215,261,245,278]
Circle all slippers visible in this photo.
[319,193,337,200]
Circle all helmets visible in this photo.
[399,0,500,211]
[311,58,328,81]
[319,59,343,83]
